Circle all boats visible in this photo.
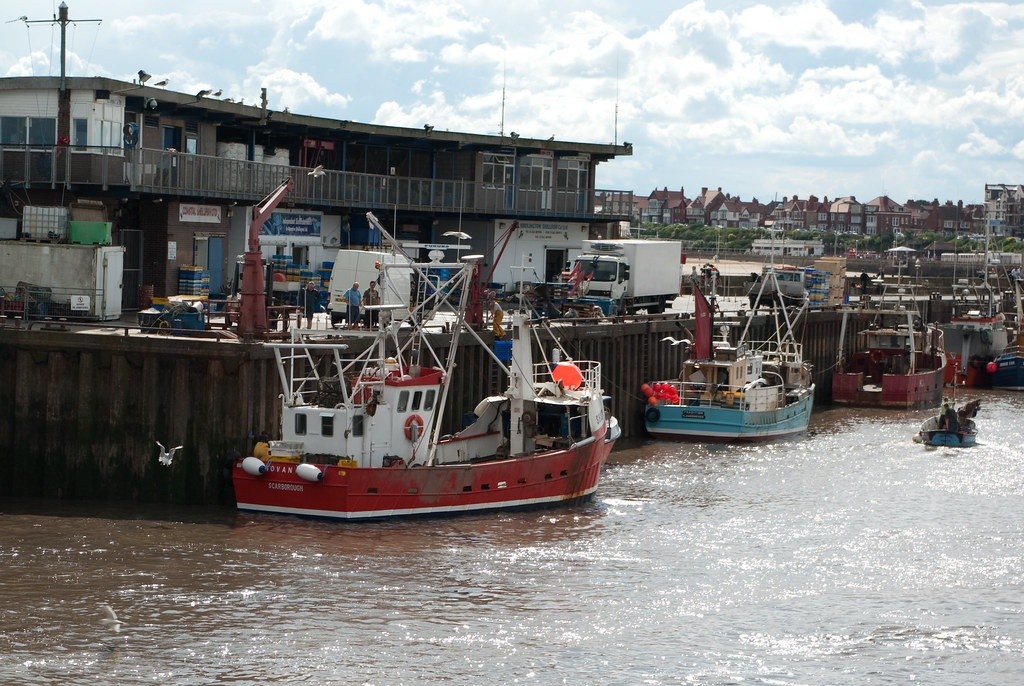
[988,274,1024,392]
[229,210,624,523]
[829,245,950,411]
[643,265,820,441]
[919,364,983,448]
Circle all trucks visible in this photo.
[556,238,685,313]
[328,249,441,325]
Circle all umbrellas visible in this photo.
[888,246,916,251]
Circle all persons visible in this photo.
[297,281,321,330]
[1011,265,1024,283]
[564,304,579,318]
[939,397,948,429]
[860,269,867,294]
[701,263,719,280]
[342,282,362,326]
[588,302,605,324]
[766,366,778,385]
[362,281,379,327]
[489,297,506,339]
[748,272,761,282]
[687,266,698,296]
[877,268,884,278]
[944,404,958,432]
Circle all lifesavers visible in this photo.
[405,414,423,439]
[766,366,779,378]
[871,350,884,363]
[971,355,981,369]
[646,408,660,422]
